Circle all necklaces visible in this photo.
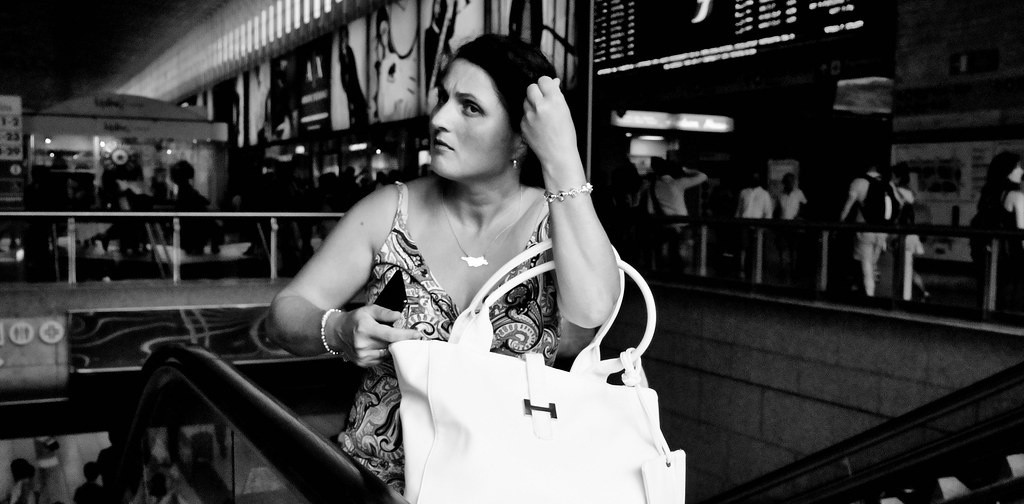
[439,179,523,268]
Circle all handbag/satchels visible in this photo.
[388,235,685,504]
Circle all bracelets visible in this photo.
[320,308,345,356]
[543,183,594,203]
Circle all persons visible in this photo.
[592,151,1024,312]
[269,33,621,497]
[0,426,141,504]
[97,159,434,281]
